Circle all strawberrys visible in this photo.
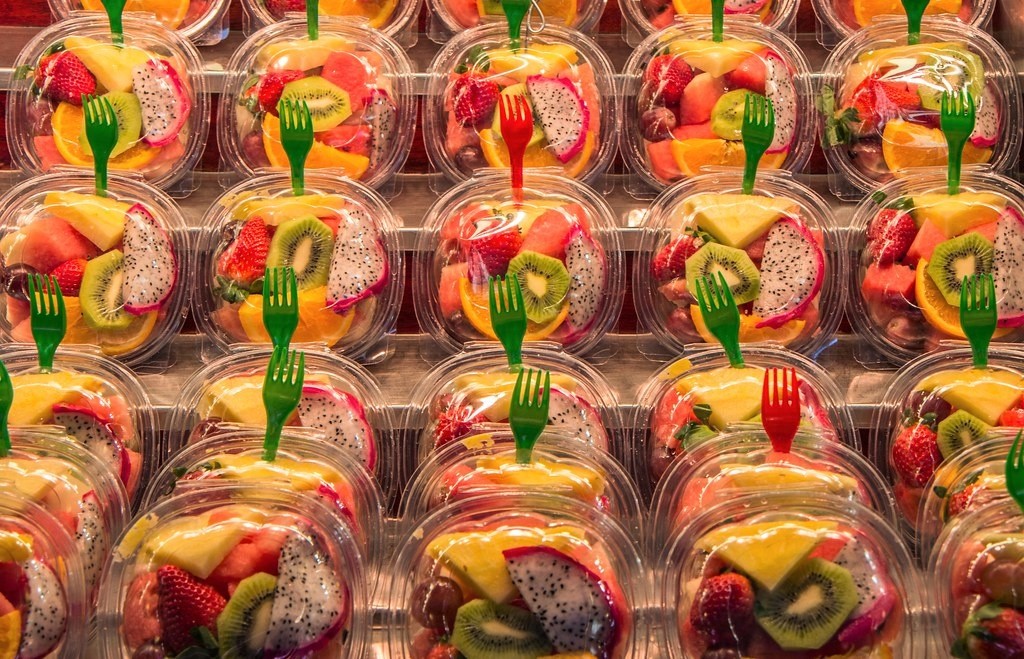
[0,52,1024,659]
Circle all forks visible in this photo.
[1004,428,1024,515]
[496,91,535,206]
[763,364,804,457]
[275,97,314,200]
[939,87,975,193]
[261,346,304,463]
[487,277,527,364]
[960,273,999,369]
[507,367,551,462]
[27,270,66,370]
[696,270,745,367]
[80,90,119,195]
[263,265,300,344]
[740,90,777,194]
